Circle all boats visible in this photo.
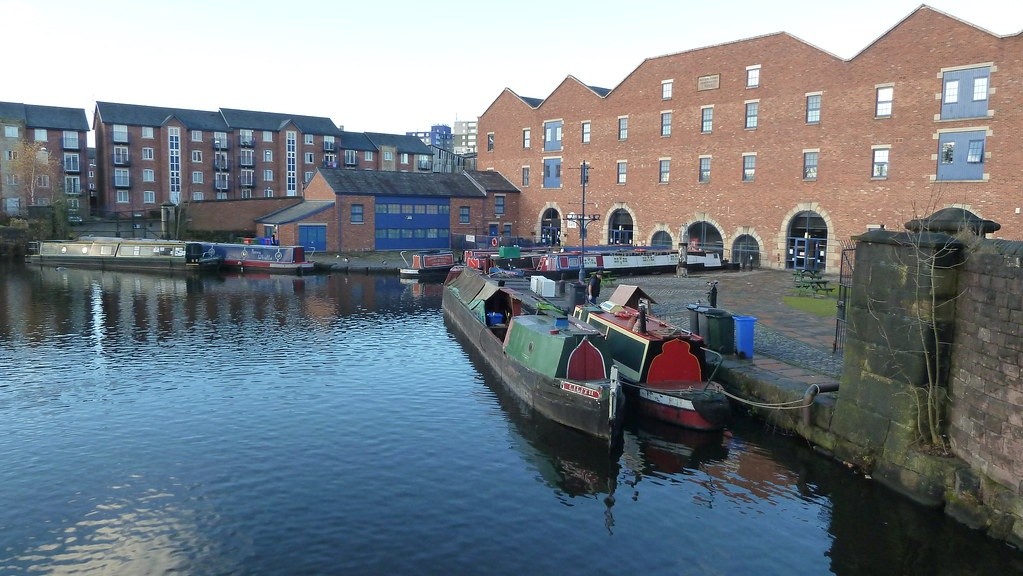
[77,236,318,274]
[400,245,722,280]
[442,265,626,453]
[25,239,224,270]
[573,300,735,437]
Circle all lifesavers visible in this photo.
[614,311,640,317]
[635,248,646,252]
[492,237,498,247]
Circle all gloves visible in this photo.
[589,295,593,300]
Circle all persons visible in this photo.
[587,269,604,304]
[270,231,276,246]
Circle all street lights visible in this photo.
[562,160,601,281]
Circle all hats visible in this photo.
[595,271,604,275]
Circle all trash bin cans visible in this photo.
[695,307,725,348]
[706,313,734,355]
[686,304,709,336]
[733,316,757,359]
